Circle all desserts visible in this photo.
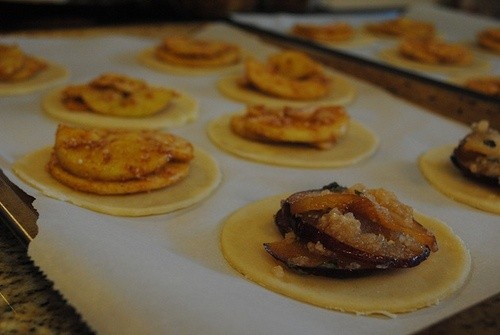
[0,18,500,320]
